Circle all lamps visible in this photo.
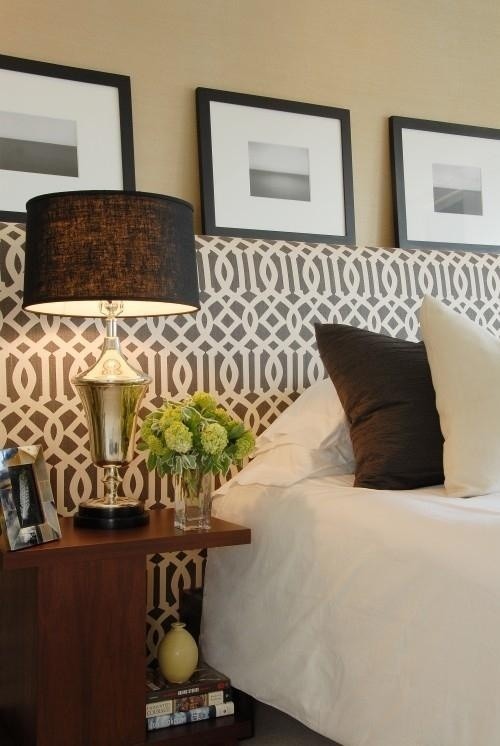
[21,190,200,530]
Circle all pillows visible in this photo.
[251,379,349,449]
[233,445,356,489]
[421,295,500,499]
[313,322,445,489]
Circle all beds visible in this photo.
[198,466,500,746]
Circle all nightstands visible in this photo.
[0,507,255,746]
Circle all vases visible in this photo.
[173,465,214,531]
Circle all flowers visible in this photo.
[138,390,259,498]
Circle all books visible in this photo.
[146,656,234,732]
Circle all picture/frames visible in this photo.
[0,54,135,221]
[0,444,62,551]
[388,114,500,254]
[195,88,356,246]
